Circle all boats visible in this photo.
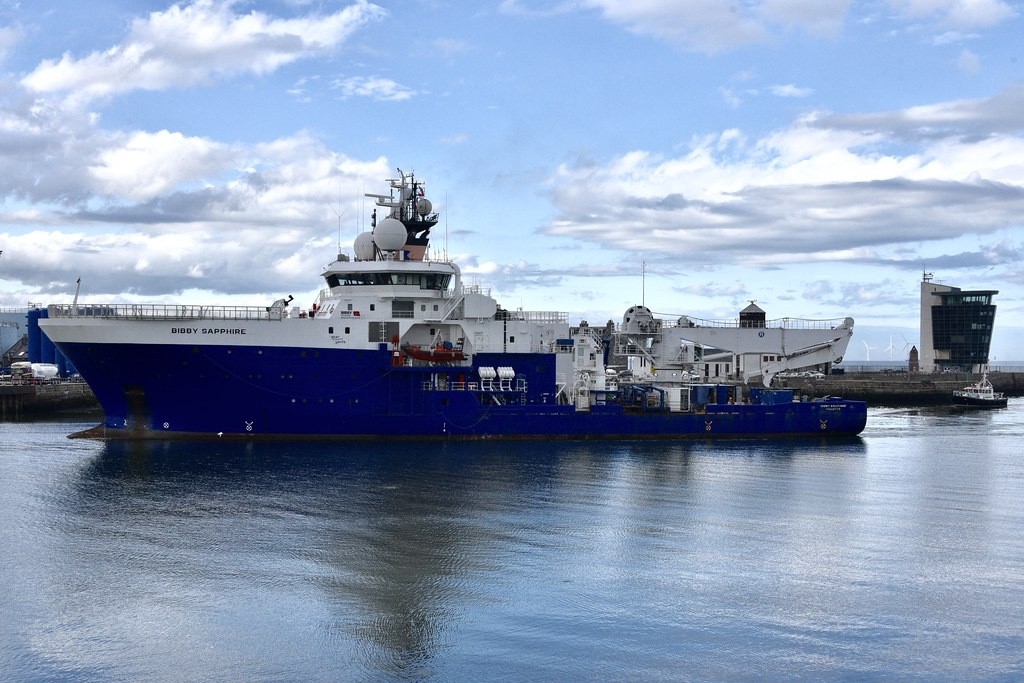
[36,164,871,443]
[401,344,467,361]
[953,371,1008,407]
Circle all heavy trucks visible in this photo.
[10,361,61,384]
